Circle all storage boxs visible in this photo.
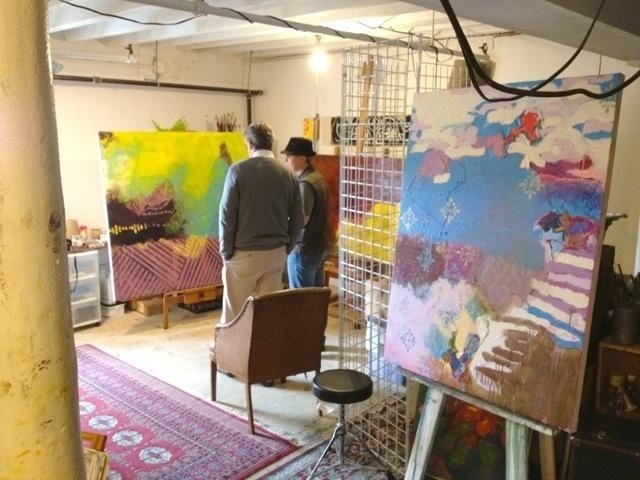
[594,335,640,419]
[103,266,170,317]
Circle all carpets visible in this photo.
[256,398,409,480]
[75,343,301,480]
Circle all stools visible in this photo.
[306,370,394,480]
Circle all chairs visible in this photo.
[210,287,333,434]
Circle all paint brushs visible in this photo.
[214,111,237,132]
[609,263,640,309]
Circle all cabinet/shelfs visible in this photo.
[68,250,101,329]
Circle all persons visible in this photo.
[279,136,330,352]
[216,121,306,387]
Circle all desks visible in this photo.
[565,431,640,480]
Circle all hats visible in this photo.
[279,136,317,156]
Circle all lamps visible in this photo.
[308,34,330,71]
[126,44,137,63]
